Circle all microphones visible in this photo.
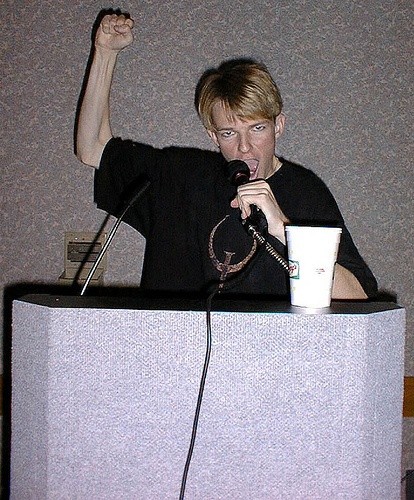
[80,177,158,296]
[224,160,269,235]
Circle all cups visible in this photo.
[286,226,341,309]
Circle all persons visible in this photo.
[77,15,378,298]
